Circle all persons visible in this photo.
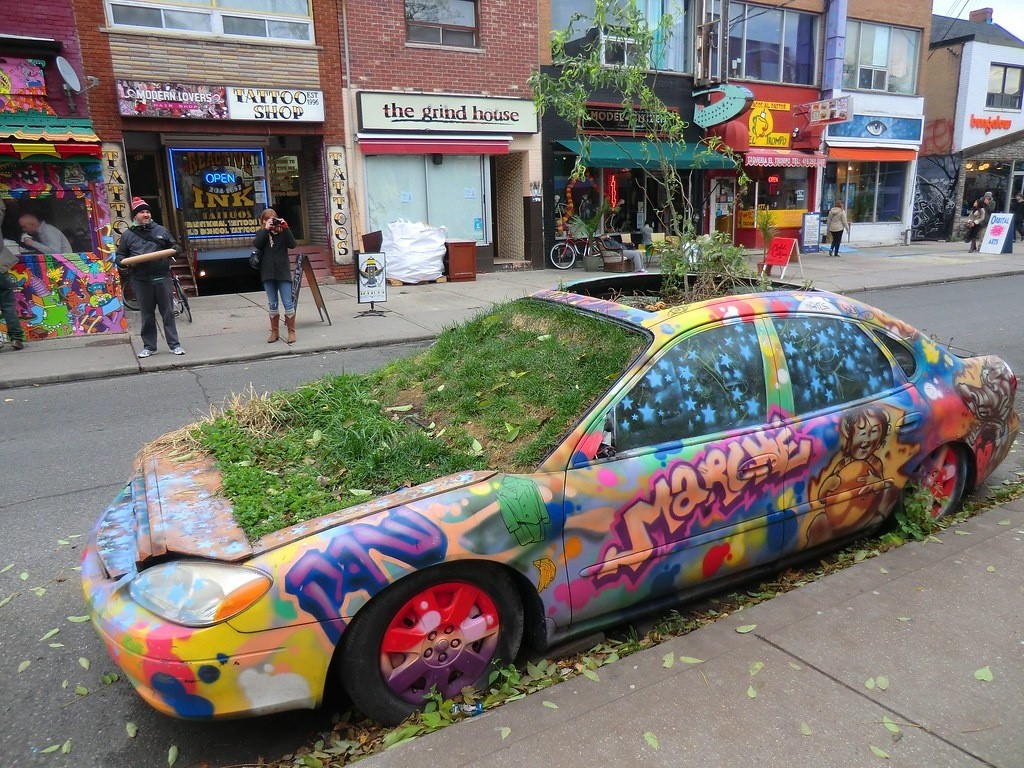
[0,196,24,349]
[978,192,995,253]
[1012,193,1024,242]
[253,209,297,343]
[967,199,985,253]
[827,200,850,257]
[116,196,186,358]
[18,213,73,254]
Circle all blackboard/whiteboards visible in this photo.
[291,256,303,314]
[980,213,1013,254]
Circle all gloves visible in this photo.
[172,244,181,254]
[115,254,124,268]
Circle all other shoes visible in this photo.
[829,249,833,256]
[0,341,4,349]
[1021,236,1024,241]
[834,254,841,257]
[1012,239,1015,242]
[638,268,648,273]
[11,339,23,349]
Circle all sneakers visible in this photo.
[170,347,185,355]
[137,349,158,358]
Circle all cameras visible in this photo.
[270,218,281,226]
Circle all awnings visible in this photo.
[554,139,738,170]
[743,148,826,168]
[0,125,102,143]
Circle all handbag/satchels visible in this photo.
[964,222,979,243]
[826,234,832,243]
[249,244,264,269]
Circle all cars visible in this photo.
[78,272,1020,726]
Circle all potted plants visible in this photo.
[561,196,612,273]
[752,203,780,276]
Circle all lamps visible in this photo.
[792,127,805,142]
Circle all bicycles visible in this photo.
[550,225,601,270]
[121,252,192,323]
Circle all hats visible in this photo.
[132,197,153,220]
[984,192,992,198]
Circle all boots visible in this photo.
[268,314,279,343]
[284,314,295,343]
[969,239,976,252]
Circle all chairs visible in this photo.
[645,233,681,268]
[595,234,636,273]
